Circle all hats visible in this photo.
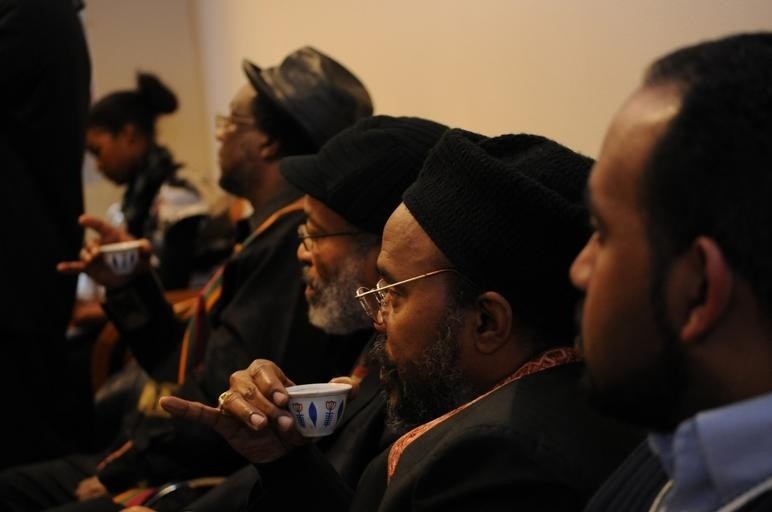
[243,47,372,153]
[403,127,593,314]
[279,117,451,248]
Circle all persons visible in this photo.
[564,25,771,512]
[279,112,450,338]
[1,2,90,470]
[153,128,645,508]
[59,68,205,437]
[1,47,373,510]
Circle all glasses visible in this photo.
[215,108,254,129]
[352,269,465,324]
[294,224,358,252]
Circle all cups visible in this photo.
[286,383,354,438]
[99,240,140,276]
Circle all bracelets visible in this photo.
[218,389,227,406]
